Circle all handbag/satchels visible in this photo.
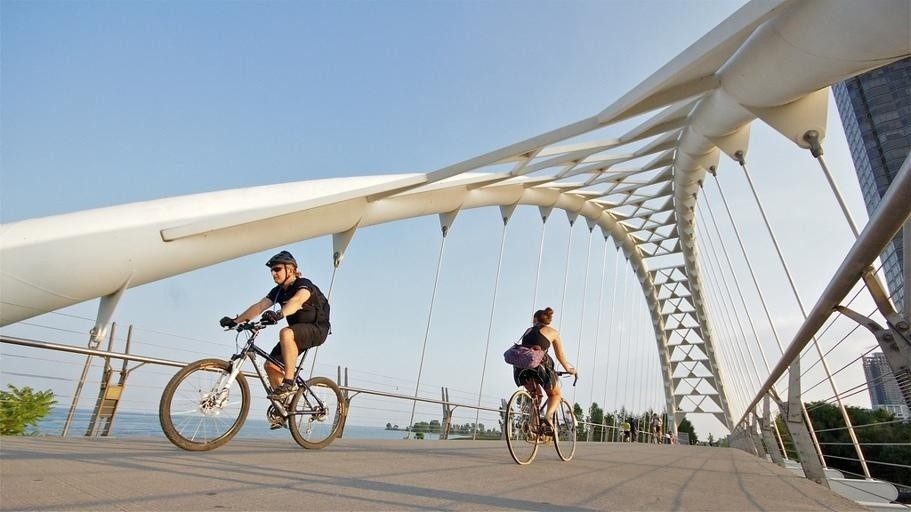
[505,344,544,369]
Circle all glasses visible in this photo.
[271,267,282,272]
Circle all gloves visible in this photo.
[259,310,284,323]
[220,316,238,327]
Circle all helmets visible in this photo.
[266,251,297,267]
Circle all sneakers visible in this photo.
[270,415,286,429]
[267,382,298,400]
[543,415,554,428]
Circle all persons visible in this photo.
[652,412,664,442]
[624,419,633,442]
[669,430,675,444]
[708,432,715,447]
[218,250,331,430]
[628,417,638,442]
[513,307,579,435]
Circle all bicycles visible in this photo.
[159,317,348,450]
[503,370,583,467]
[650,425,665,449]
